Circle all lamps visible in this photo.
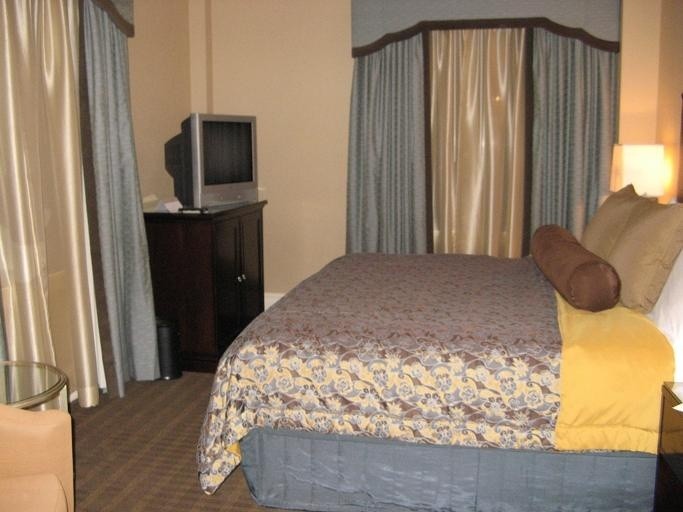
[608,142,664,198]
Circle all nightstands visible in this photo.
[651,380,681,512]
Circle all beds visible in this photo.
[194,185,682,512]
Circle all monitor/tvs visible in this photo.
[164,112,259,209]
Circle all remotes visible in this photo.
[179,208,209,214]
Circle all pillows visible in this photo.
[529,219,621,312]
[641,248,682,382]
[604,194,681,314]
[578,180,657,259]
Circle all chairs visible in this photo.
[0,404,76,512]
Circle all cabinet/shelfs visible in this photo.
[142,197,268,373]
